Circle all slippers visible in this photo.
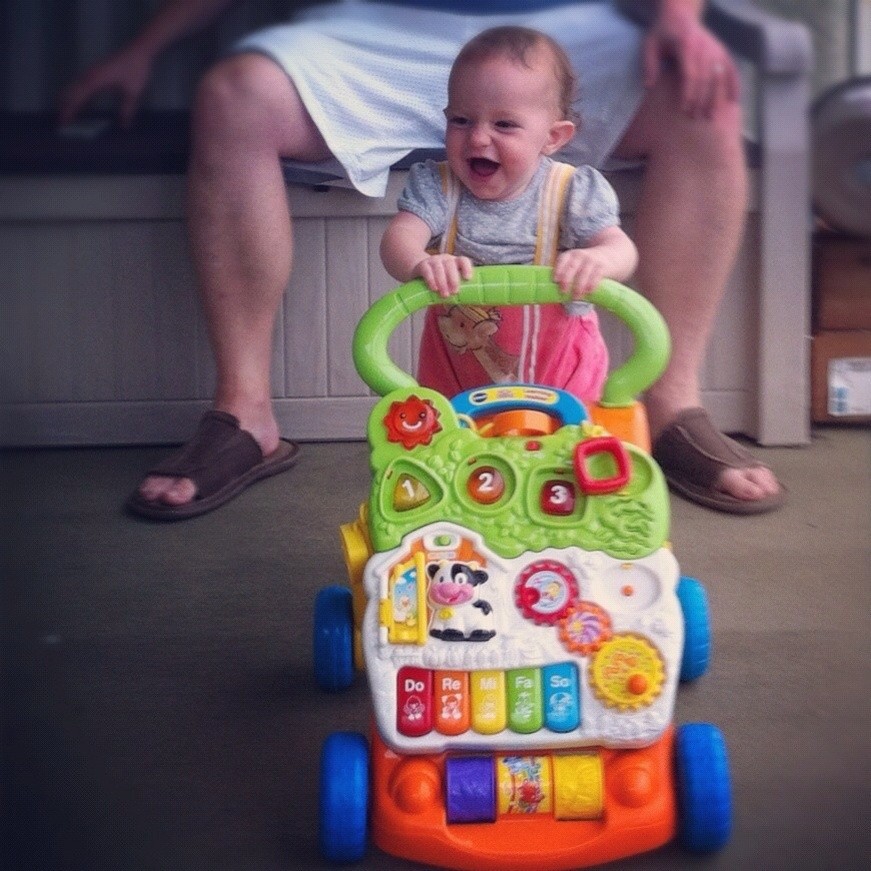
[125,410,301,522]
[652,410,786,516]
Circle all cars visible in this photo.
[314,262,734,871]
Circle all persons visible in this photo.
[380,26,641,407]
[60,0,789,523]
